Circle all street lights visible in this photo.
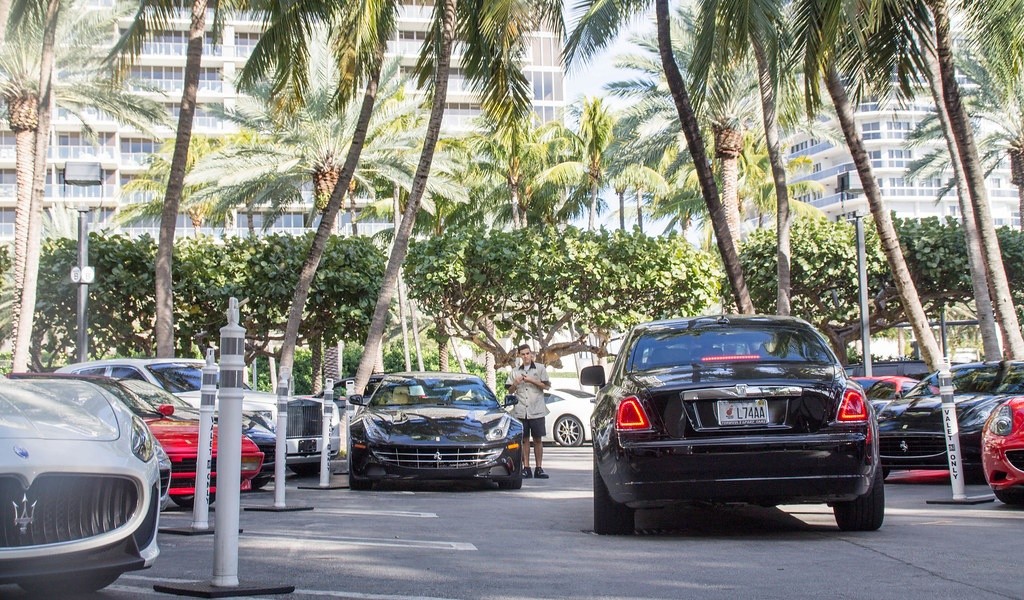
[835,170,873,376]
[60,163,106,363]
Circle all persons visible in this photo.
[504,344,551,479]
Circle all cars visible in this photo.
[844,361,939,418]
[348,371,524,489]
[874,360,1024,484]
[581,314,890,536]
[982,395,1024,504]
[528,387,595,447]
[317,375,384,416]
[0,374,172,600]
[52,358,339,477]
[106,376,265,509]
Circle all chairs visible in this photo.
[387,386,414,404]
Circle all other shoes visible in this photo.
[534,468,549,478]
[521,467,532,478]
